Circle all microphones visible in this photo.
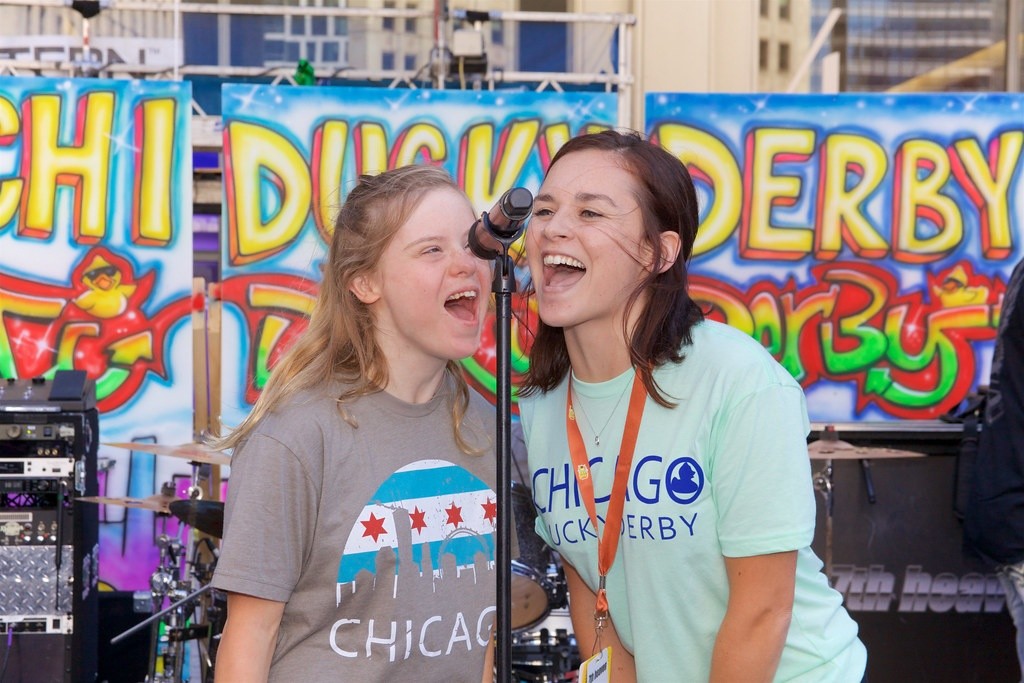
[468,187,534,261]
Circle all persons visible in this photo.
[208,166,530,683]
[518,132,876,681]
